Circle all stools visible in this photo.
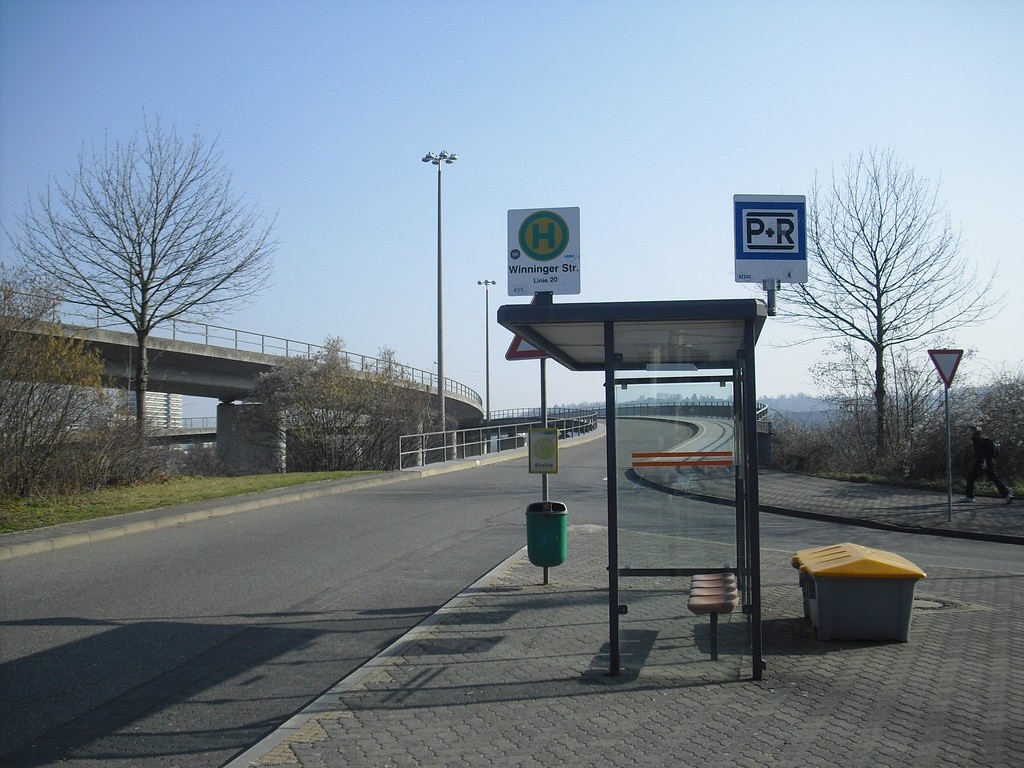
[691,573,735,580]
[688,594,739,661]
[689,587,739,596]
[691,580,737,587]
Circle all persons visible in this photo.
[957,425,1014,506]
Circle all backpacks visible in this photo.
[974,436,1001,457]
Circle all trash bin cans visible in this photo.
[525,501,568,569]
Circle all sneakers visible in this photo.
[1005,494,1014,504]
[960,496,975,502]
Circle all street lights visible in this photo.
[478,280,496,454]
[420,148,458,432]
